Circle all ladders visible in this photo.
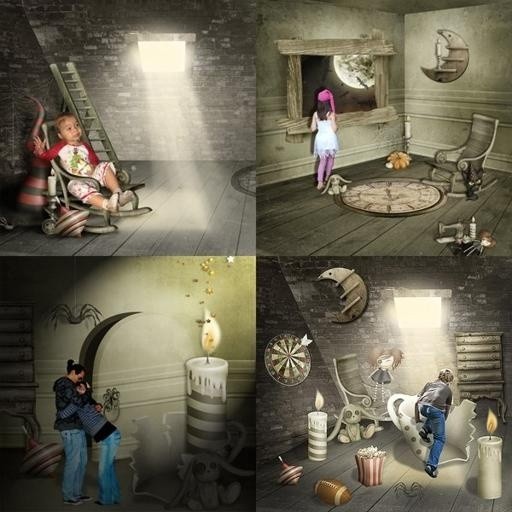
[49,62,131,185]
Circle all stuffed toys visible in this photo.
[168,452,256,512]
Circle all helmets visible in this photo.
[439,369,454,383]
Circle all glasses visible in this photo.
[76,373,81,380]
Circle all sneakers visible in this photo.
[106,190,134,213]
[64,496,118,506]
[317,181,327,190]
[425,464,437,478]
[419,429,430,443]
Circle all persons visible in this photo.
[415,367,454,477]
[53,364,104,505]
[56,381,123,506]
[32,113,134,211]
[310,88,339,190]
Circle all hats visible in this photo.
[80,381,92,390]
[318,89,335,112]
